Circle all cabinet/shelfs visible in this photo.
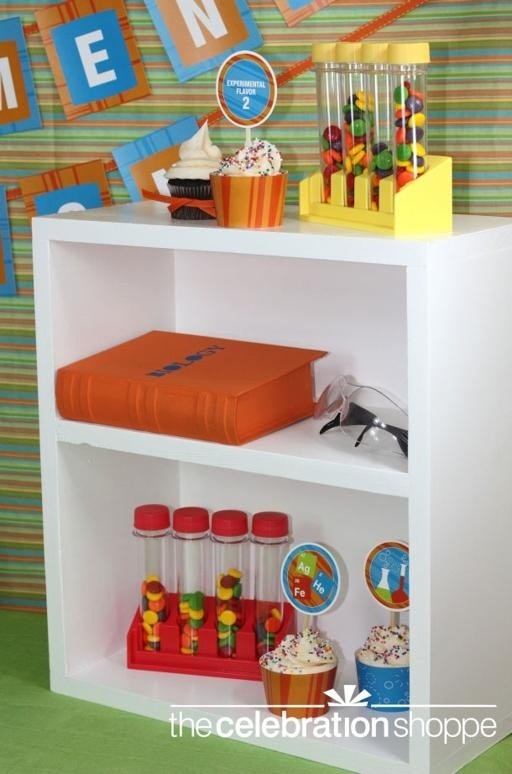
[24,193,510,773]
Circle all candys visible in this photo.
[320,78,427,212]
[139,571,281,660]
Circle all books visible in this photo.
[52,329,326,446]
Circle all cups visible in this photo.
[255,657,340,718]
[208,171,291,229]
[166,177,216,220]
[354,655,411,712]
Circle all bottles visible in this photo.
[130,501,293,660]
[312,36,429,206]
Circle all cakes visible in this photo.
[166,118,223,218]
[256,629,338,719]
[206,136,290,227]
[355,623,409,714]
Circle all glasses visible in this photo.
[314,375,408,457]
